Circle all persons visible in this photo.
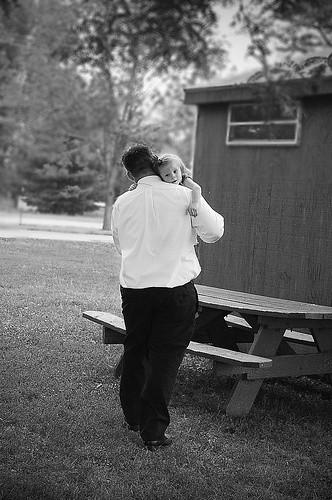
[111,144,225,446]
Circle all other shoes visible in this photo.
[128,424,141,433]
[144,439,173,450]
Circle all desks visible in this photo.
[192,285,332,417]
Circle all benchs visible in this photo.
[221,314,313,368]
[82,311,272,379]
